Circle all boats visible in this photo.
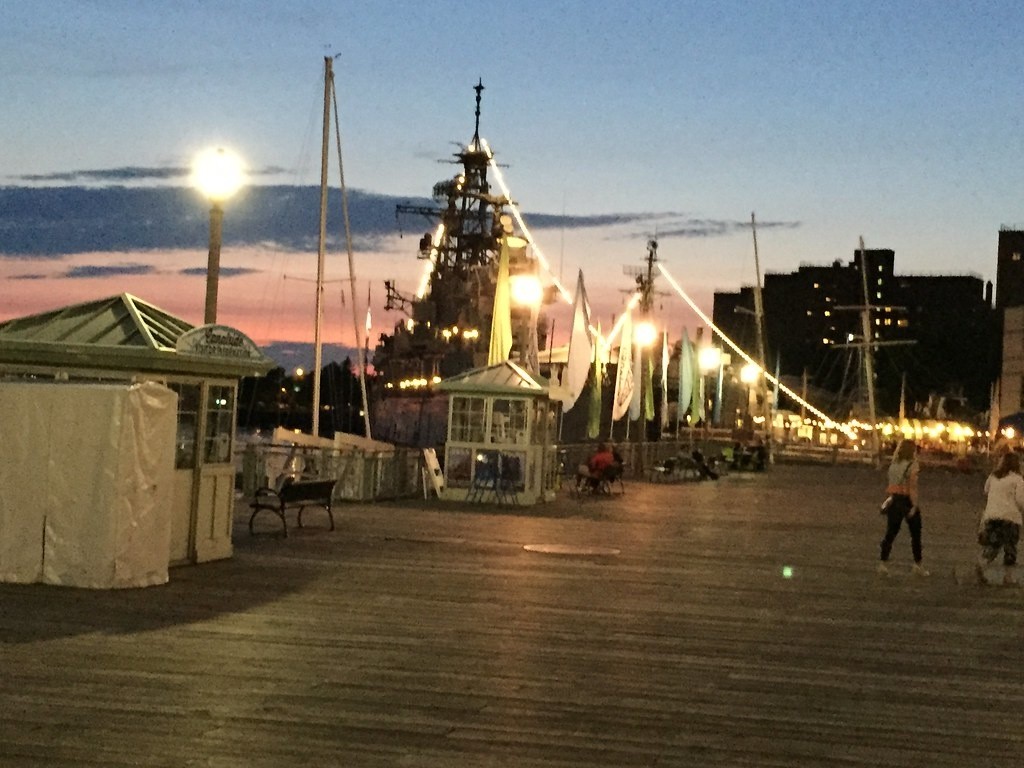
[367,74,549,473]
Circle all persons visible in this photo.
[975,453,1024,587]
[881,437,1024,463]
[580,432,772,496]
[875,439,931,577]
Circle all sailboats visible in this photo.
[229,51,396,488]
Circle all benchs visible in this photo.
[249,480,337,537]
[575,462,625,500]
[642,458,677,486]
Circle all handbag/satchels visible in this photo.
[977,510,989,546]
[880,496,892,514]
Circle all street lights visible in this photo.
[189,144,245,324]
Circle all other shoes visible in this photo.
[974,567,988,585]
[876,560,888,577]
[1001,576,1021,589]
[912,561,929,577]
